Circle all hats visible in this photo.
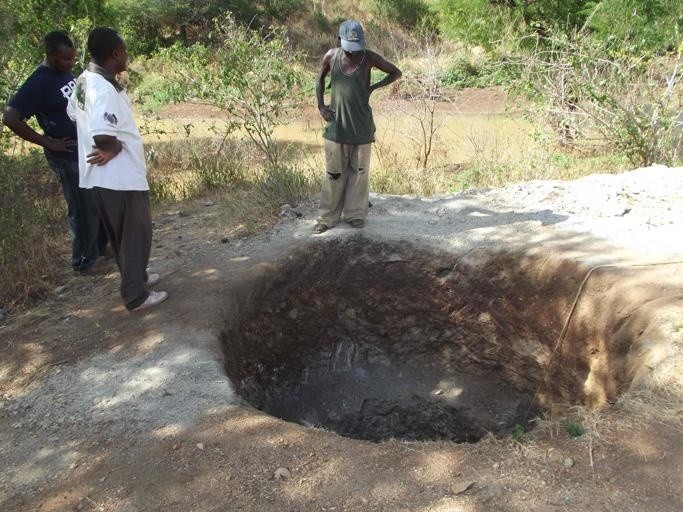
[337,19,367,54]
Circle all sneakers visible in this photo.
[101,246,115,257]
[72,254,113,274]
[129,290,169,313]
[146,273,160,287]
[312,223,328,234]
[349,219,365,228]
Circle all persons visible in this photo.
[1,28,114,275]
[65,26,170,314]
[310,18,401,233]
